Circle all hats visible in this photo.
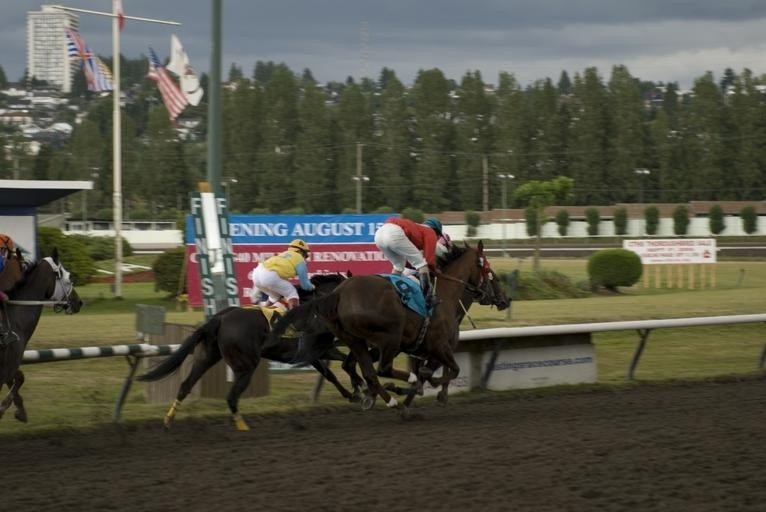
[288,240,310,252]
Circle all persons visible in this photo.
[404,231,456,278]
[249,239,316,315]
[0,233,15,304]
[371,215,444,310]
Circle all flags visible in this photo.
[145,41,189,122]
[65,23,113,92]
[163,34,204,107]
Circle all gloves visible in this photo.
[0,291,9,301]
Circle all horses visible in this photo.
[122,267,362,432]
[0,245,83,424]
[257,240,513,421]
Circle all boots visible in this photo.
[420,273,444,308]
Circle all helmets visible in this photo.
[0,234,14,248]
[424,217,442,236]
